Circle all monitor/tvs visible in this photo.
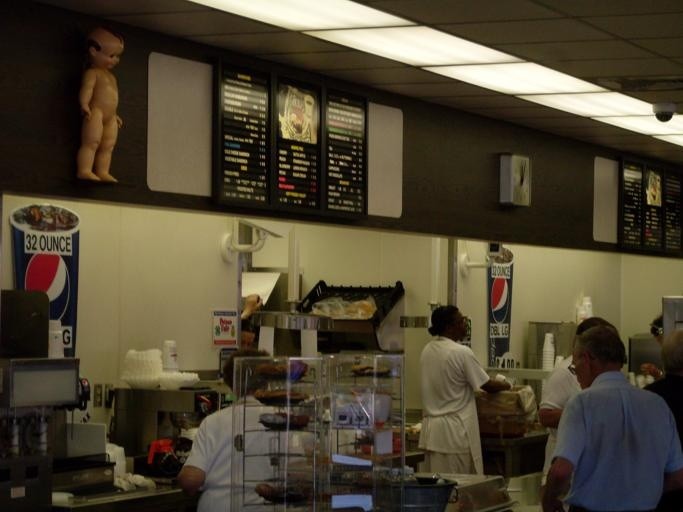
[9,358,80,408]
[0,290,50,358]
[662,295,683,336]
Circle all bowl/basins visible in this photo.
[385,476,458,511]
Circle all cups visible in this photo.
[541,333,565,371]
[486,248,516,363]
[162,341,179,374]
[10,203,81,358]
[304,95,314,117]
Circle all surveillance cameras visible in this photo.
[487,241,502,256]
[653,102,676,122]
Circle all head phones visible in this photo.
[650,324,663,336]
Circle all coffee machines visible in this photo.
[111,388,194,477]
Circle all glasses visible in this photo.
[568,360,576,375]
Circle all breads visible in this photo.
[255,483,304,502]
[260,414,309,426]
[258,362,308,375]
[255,390,304,399]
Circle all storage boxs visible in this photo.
[475,383,538,437]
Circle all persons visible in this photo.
[75,25,125,183]
[639,315,664,380]
[646,169,662,207]
[176,349,308,512]
[641,327,683,512]
[541,324,683,512]
[537,316,618,484]
[420,304,513,476]
